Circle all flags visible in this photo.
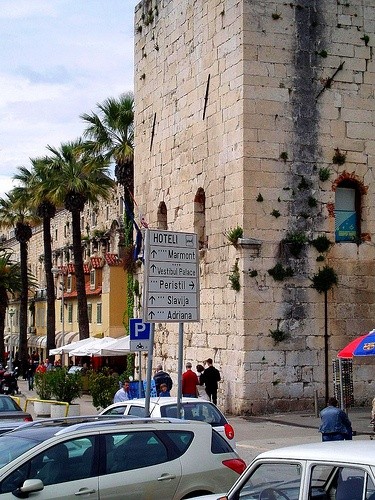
[127,208,142,261]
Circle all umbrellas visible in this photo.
[49,334,135,357]
[336,328,375,359]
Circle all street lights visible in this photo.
[51,267,65,373]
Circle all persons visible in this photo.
[182,362,198,399]
[319,397,352,441]
[159,383,170,397]
[196,365,209,402]
[114,380,130,414]
[153,364,172,397]
[0,351,52,395]
[200,358,220,405]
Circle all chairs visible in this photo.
[335,479,364,500]
[84,435,150,472]
[185,407,193,419]
[167,408,178,419]
[35,444,68,485]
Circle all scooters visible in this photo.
[0,365,20,395]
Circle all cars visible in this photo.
[178,439,375,500]
[0,394,33,435]
[95,396,238,455]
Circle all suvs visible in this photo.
[0,413,248,500]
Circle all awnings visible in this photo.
[3,330,80,348]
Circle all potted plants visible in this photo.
[32,366,83,418]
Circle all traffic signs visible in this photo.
[147,292,200,308]
[149,245,199,263]
[147,261,200,277]
[150,230,198,249]
[147,308,200,323]
[147,275,200,292]
